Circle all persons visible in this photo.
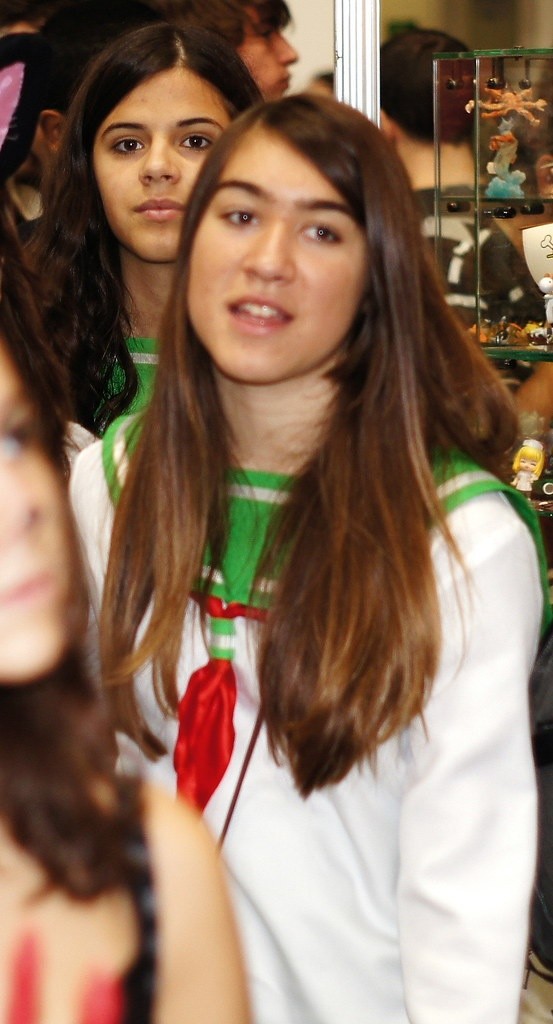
[118,0,305,120]
[32,34,275,507]
[20,24,126,224]
[379,27,513,321]
[1,307,258,1024]
[440,107,553,434]
[56,84,553,1024]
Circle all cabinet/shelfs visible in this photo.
[432,45,552,603]
